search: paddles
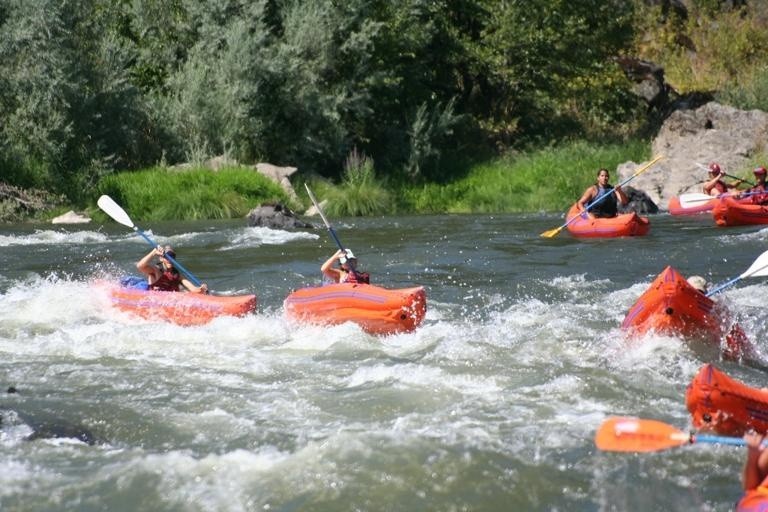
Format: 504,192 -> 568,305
696,163 -> 756,186
540,156 -> 663,238
594,416 -> 768,453
679,190 -> 767,209
98,194 -> 210,294
304,182 -> 358,276
701,251 -> 768,296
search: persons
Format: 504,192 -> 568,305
701,162 -> 745,198
743,429 -> 768,489
136,244 -> 209,294
578,168 -> 629,219
321,248 -> 369,285
685,275 -> 708,297
734,166 -> 768,205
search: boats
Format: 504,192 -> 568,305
93,278 -> 258,326
719,323 -> 768,374
566,200 -> 651,242
618,266 -> 730,339
283,281 -> 428,335
713,194 -> 768,229
685,363 -> 768,439
736,474 -> 768,512
668,189 -> 747,215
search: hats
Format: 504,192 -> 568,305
339,248 -> 357,265
752,167 -> 767,176
687,275 -> 707,292
708,163 -> 720,172
161,244 -> 177,257
